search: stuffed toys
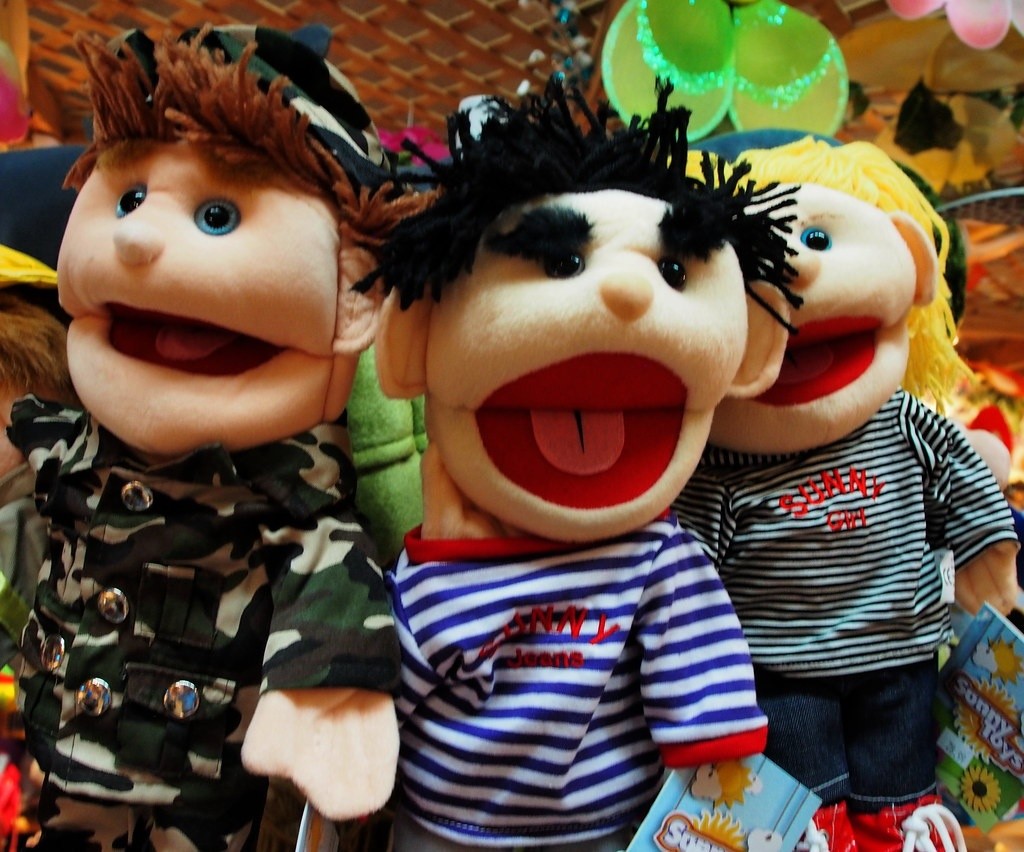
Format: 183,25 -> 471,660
0,21 -> 805,852
668,134 -> 1024,852
